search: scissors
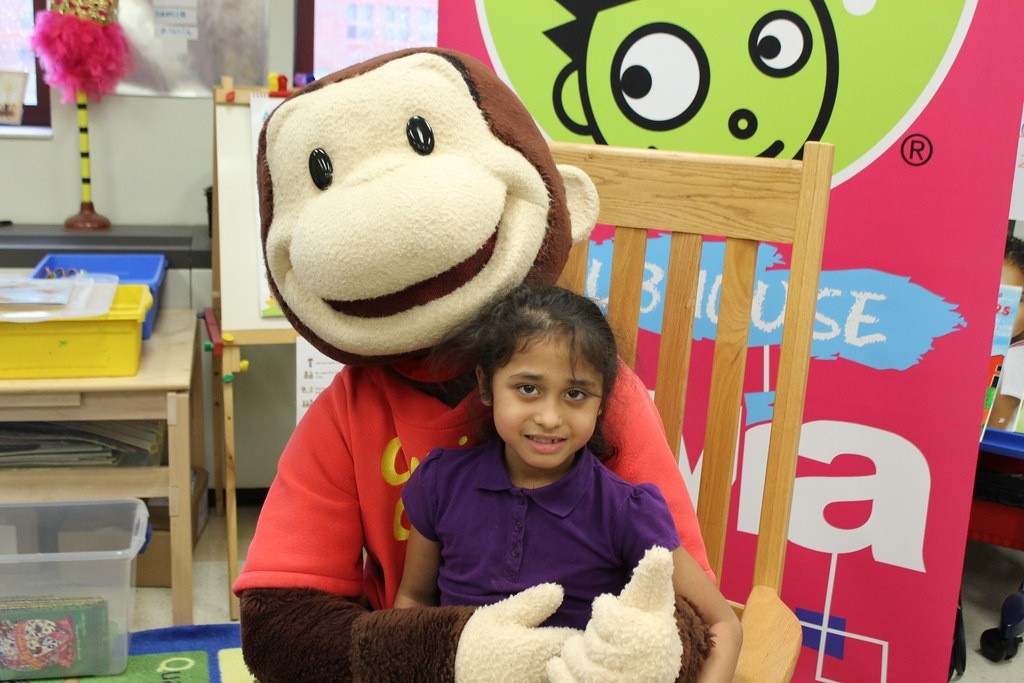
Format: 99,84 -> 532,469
55,267 -> 78,280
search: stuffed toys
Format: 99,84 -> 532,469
232,46 -> 719,683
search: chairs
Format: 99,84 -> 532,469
546,139 -> 835,683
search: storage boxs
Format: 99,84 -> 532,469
28,253 -> 169,340
0,464 -> 210,588
0,497 -> 152,682
0,283 -> 155,380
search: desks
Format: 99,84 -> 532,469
0,306 -> 205,627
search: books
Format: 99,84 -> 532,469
0,420 -> 164,470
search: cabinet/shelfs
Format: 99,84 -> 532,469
0,225 -> 296,489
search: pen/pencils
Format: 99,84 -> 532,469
46,267 -> 55,279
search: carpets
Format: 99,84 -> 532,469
2,623 -> 258,683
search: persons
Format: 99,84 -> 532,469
394,287 -> 742,683
